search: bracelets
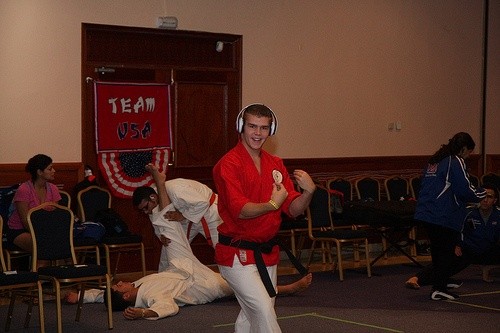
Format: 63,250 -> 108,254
142,309 -> 144,318
269,200 -> 279,210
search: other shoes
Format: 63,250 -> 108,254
430,290 -> 460,301
448,281 -> 463,289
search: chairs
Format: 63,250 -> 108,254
280,175 -> 500,281
0,183 -> 147,333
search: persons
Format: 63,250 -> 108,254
214,103 -> 315,332
6,154 -> 64,303
66,162 -> 315,321
133,178 -> 223,271
405,132 -> 500,299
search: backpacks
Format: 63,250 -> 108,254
73,218 -> 106,246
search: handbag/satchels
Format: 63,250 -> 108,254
95,207 -> 128,236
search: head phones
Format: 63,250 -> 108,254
235,103 -> 279,137
